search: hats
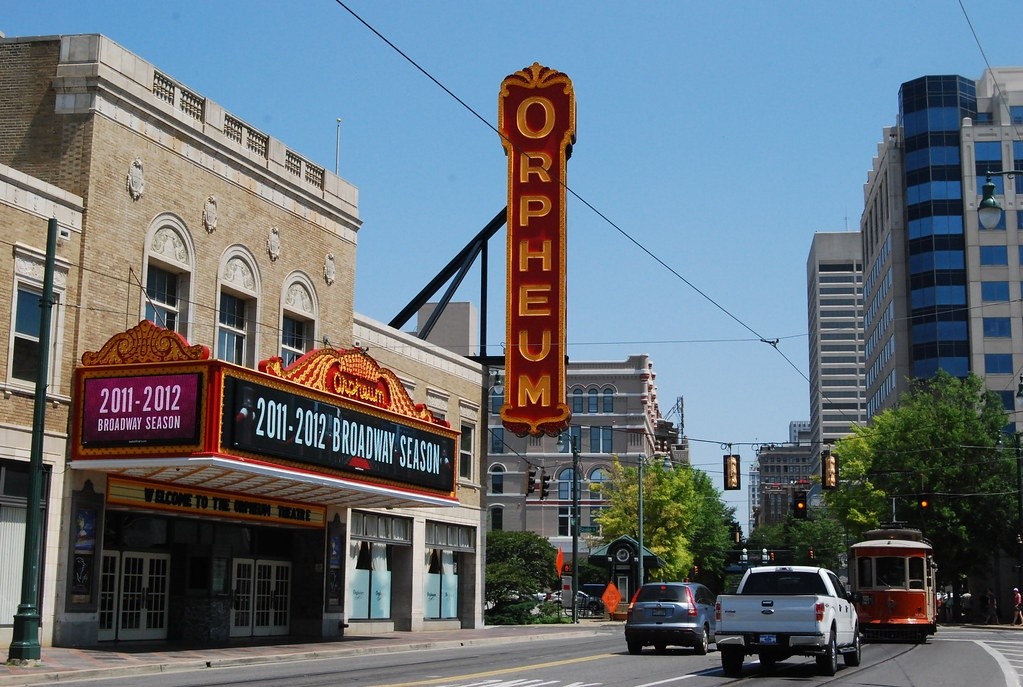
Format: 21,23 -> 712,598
1012,588 -> 1019,593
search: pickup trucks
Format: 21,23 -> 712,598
714,564 -> 867,673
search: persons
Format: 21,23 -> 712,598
1011,588 -> 1023,626
984,588 -> 999,624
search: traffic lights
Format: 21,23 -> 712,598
809,550 -> 815,560
564,565 -> 570,572
770,553 -> 777,562
792,490 -> 813,521
524,469 -> 537,498
919,492 -> 936,520
539,474 -> 551,501
693,566 -> 699,576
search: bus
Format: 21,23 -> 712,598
850,497 -> 940,642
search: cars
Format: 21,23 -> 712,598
548,589 -> 607,608
625,580 -> 716,651
506,590 -> 548,603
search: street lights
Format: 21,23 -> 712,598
995,430 -> 1023,597
638,453 -> 675,588
556,432 -> 582,623
